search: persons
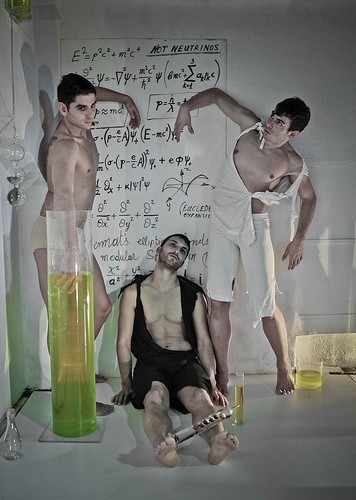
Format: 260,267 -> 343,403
174,86 -> 317,395
111,234 -> 240,468
30,73 -> 141,417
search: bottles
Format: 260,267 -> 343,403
4,409 -> 22,460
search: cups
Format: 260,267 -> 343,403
297,356 -> 324,389
